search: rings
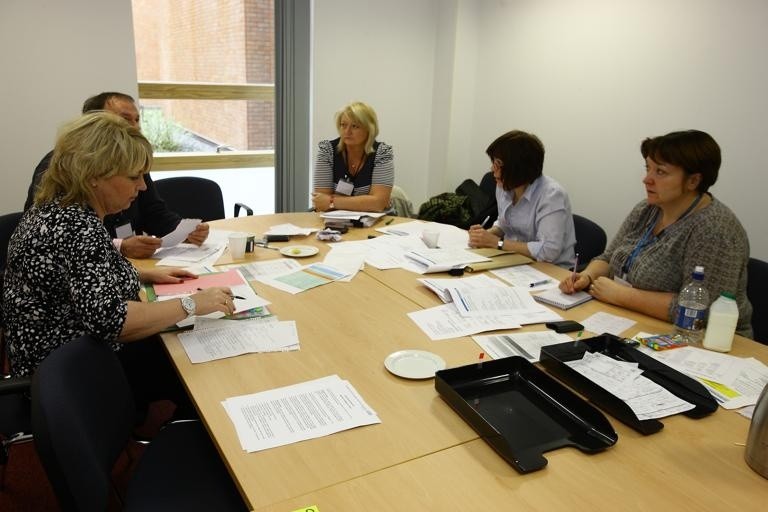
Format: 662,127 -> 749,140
591,284 -> 595,289
224,299 -> 228,305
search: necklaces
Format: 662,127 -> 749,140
351,164 -> 357,168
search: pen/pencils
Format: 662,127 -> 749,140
480,216 -> 490,228
254,239 -> 280,250
572,253 -> 579,295
529,279 -> 552,287
385,219 -> 394,225
197,288 -> 245,299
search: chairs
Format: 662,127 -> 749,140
571,212 -> 607,278
128,178 -> 254,238
744,256 -> 768,347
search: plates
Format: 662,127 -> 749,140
280,245 -> 319,258
383,350 -> 446,379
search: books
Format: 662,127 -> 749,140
533,286 -> 594,310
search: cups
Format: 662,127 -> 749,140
228,233 -> 247,258
422,229 -> 439,248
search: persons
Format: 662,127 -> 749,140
310,102 -> 395,212
467,130 -> 578,270
559,129 -> 756,339
22,91 -> 210,262
3,110 -> 240,378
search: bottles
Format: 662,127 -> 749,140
744,382 -> 767,478
702,289 -> 739,356
673,265 -> 708,344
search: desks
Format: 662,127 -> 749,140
134,210 -> 768,512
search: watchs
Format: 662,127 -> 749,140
178,295 -> 196,320
497,237 -> 504,250
329,194 -> 335,211
